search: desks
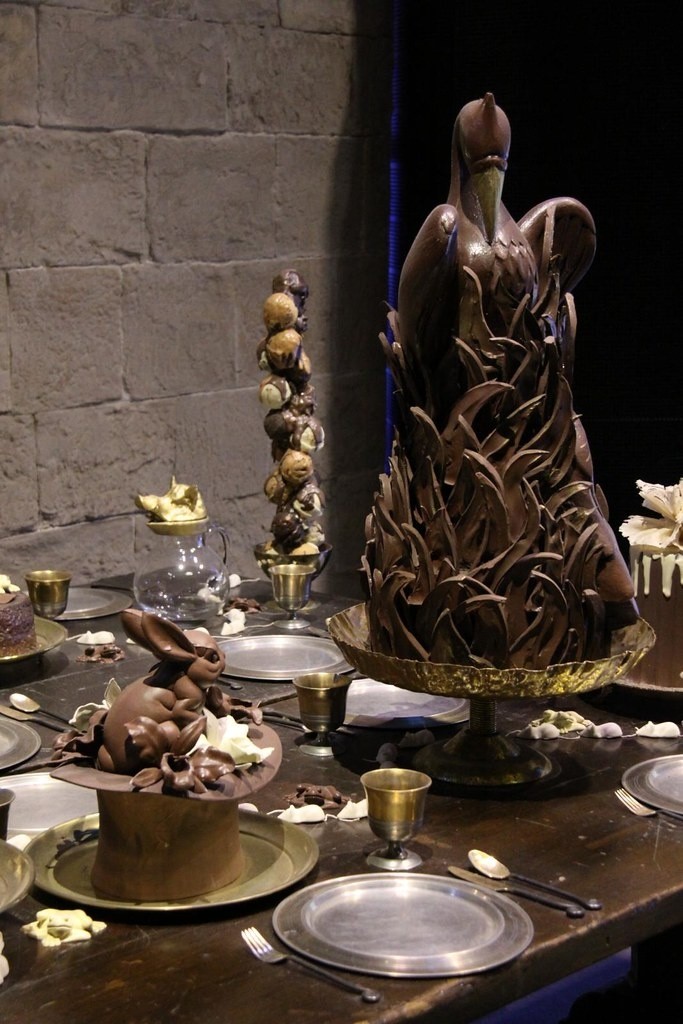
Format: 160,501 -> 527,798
1,571 -> 682,1024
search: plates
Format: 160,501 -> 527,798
343,676 -> 464,724
215,635 -> 355,681
272,872 -> 534,978
620,753 -> 683,813
0,715 -> 42,772
22,588 -> 132,621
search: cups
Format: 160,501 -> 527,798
23,569 -> 71,619
0,787 -> 16,840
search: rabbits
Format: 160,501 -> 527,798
97,609 -> 225,778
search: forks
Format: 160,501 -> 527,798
241,926 -> 381,1002
614,788 -> 683,821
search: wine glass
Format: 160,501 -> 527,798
292,672 -> 352,757
361,769 -> 432,871
269,564 -> 316,629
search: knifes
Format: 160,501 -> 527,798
0,704 -> 65,731
448,865 -> 585,917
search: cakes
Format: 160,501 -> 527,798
618,478 -> 683,689
0,574 -> 37,658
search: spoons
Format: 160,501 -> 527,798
10,693 -> 68,722
468,848 -> 602,909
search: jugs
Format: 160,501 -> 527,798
133,477 -> 231,622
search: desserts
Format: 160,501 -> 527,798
337,714 -> 434,768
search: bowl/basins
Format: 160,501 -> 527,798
0,618 -> 68,661
0,838 -> 36,914
